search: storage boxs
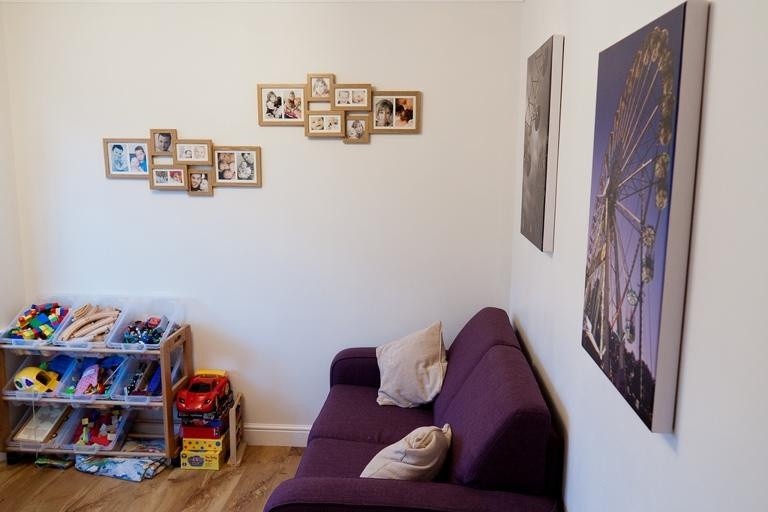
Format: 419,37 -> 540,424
52,298 -> 125,360
0,304 -> 73,356
1,353 -> 75,410
60,406 -> 138,453
112,350 -> 182,412
107,294 -> 181,360
3,405 -> 78,449
56,355 -> 127,409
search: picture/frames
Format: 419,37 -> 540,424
257,72 -> 422,144
102,129 -> 262,196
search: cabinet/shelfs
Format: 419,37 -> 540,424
0,323 -> 195,465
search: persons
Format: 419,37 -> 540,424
109,135 -> 255,192
266,79 -> 413,138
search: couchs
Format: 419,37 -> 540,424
262,307 -> 565,512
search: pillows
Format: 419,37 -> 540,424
376,319 -> 448,408
359,423 -> 452,482
